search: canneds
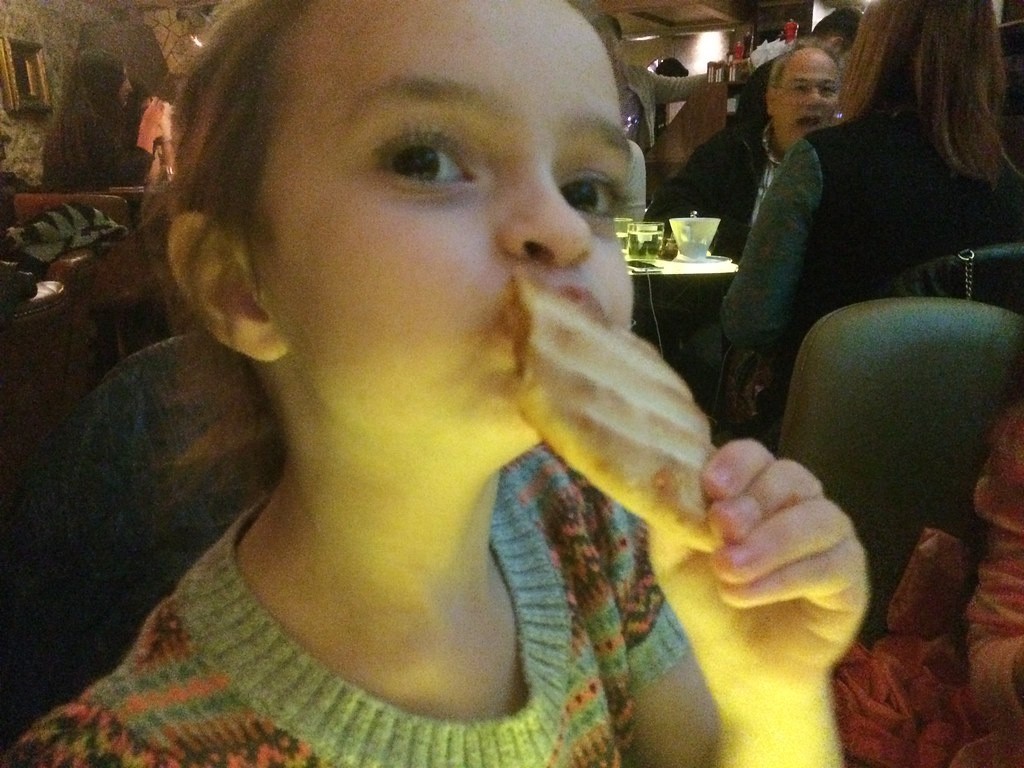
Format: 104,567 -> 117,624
707,61 -> 741,81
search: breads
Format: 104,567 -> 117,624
502,275 -> 723,553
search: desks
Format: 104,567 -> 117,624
631,235 -> 738,347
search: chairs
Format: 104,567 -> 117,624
772,290 -> 1024,651
0,190 -> 278,749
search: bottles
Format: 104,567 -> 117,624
734,41 -> 744,60
144,136 -> 173,193
784,18 -> 799,43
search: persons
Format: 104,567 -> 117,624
42,49 -> 165,187
592,15 -> 705,151
963,364 -> 1024,719
0,0 -> 870,767
721,1 -> 1024,352
734,8 -> 863,134
642,39 -> 843,261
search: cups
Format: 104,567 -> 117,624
708,60 -> 737,83
612,216 -> 634,254
626,222 -> 666,265
669,216 -> 721,260
